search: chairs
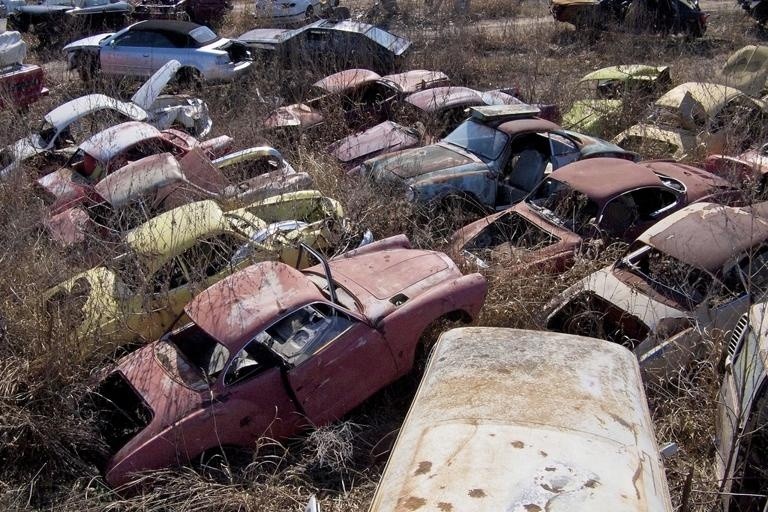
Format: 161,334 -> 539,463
499,150 -> 545,202
